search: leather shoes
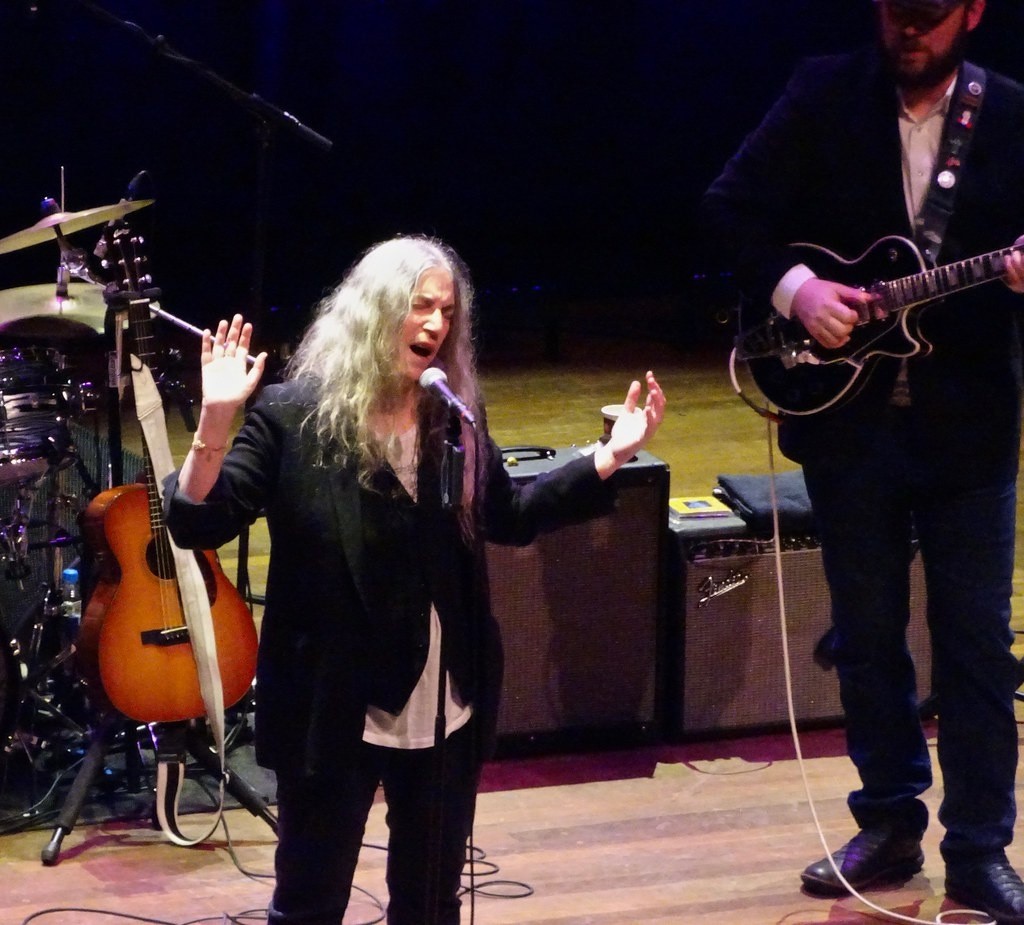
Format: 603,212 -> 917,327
941,848 -> 1024,924
797,816 -> 926,895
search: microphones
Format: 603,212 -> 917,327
418,367 -> 476,424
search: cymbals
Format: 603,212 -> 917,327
0,199 -> 156,255
0,282 -> 160,334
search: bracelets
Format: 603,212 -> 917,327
191,431 -> 230,462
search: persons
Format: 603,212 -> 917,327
670,0 -> 1024,925
162,235 -> 665,925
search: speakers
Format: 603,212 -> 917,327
480,446 -> 933,755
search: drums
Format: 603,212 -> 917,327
1,346 -> 79,487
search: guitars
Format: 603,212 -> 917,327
737,237 -> 1023,417
73,214 -> 260,724
